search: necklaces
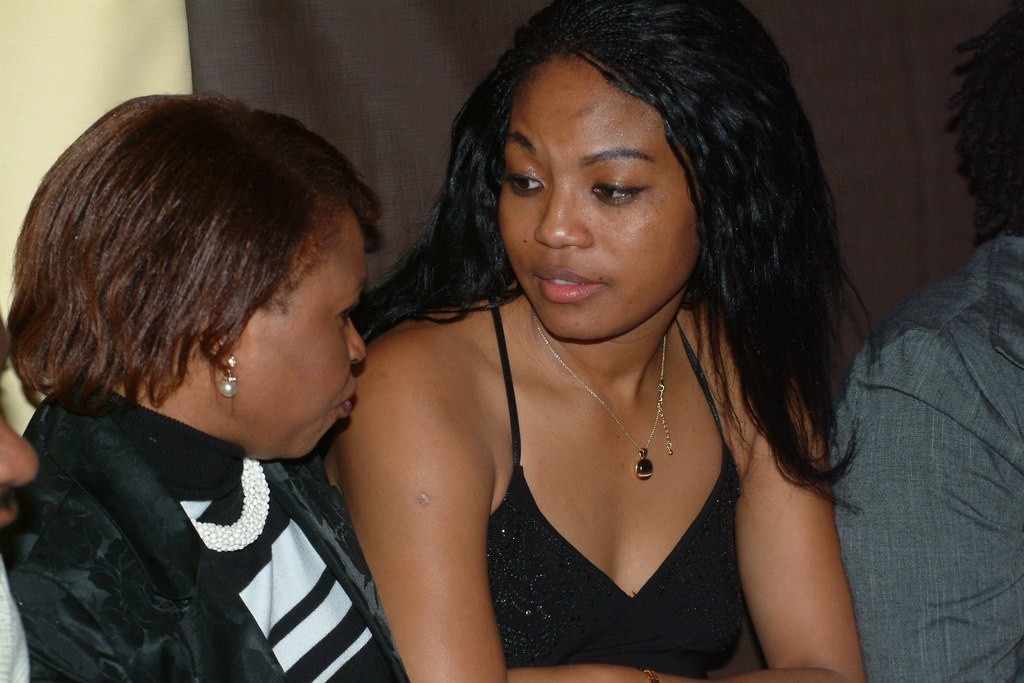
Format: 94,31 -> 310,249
532,313 -> 675,481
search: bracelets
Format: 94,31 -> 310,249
639,666 -> 661,683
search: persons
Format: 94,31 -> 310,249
0,315 -> 50,683
820,0 -> 1024,683
12,92 -> 417,683
307,0 -> 874,683
